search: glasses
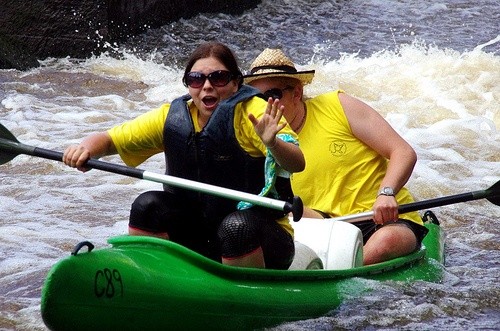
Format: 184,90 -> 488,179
184,70 -> 236,89
260,86 -> 293,102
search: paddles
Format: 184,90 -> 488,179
329,179 -> 500,222
0,123 -> 304,221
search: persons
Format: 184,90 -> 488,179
61,42 -> 306,270
242,47 -> 429,265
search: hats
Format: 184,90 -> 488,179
243,48 -> 315,86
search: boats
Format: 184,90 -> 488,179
39,209 -> 448,331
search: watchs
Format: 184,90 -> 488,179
376,186 -> 396,198
